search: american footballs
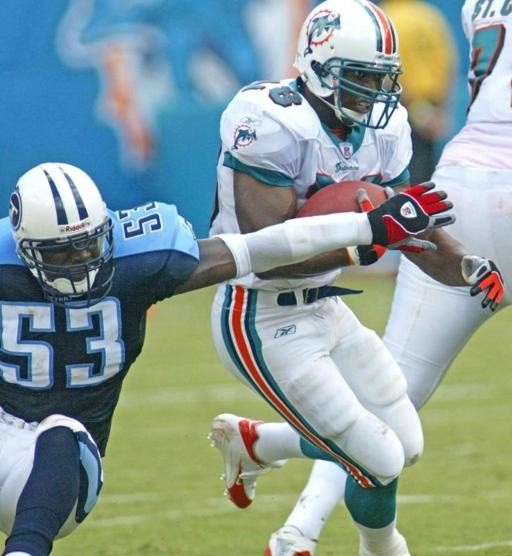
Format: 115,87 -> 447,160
294,181 -> 387,218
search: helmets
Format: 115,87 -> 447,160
294,0 -> 399,98
10,163 -> 108,297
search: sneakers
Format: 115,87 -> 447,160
265,533 -> 316,556
208,414 -> 287,509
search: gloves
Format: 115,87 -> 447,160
461,255 -> 505,312
346,180 -> 456,265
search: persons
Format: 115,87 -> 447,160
1,161 -> 455,556
211,1 -> 503,556
264,1 -> 512,555
